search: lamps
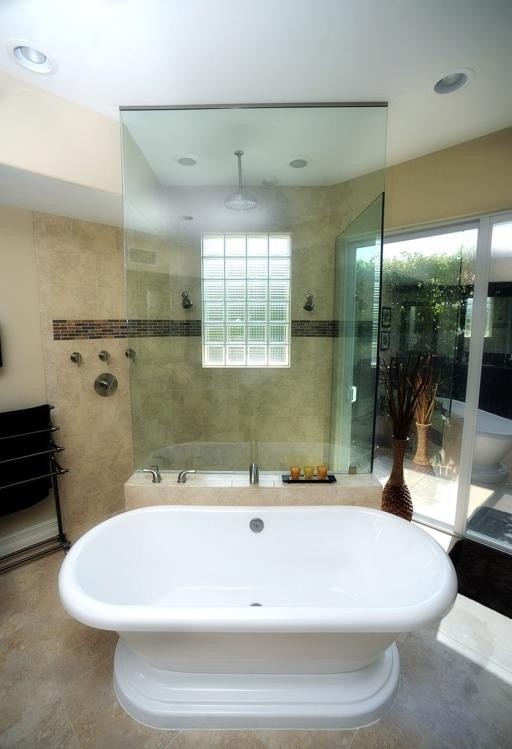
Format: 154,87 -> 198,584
224,138 -> 258,212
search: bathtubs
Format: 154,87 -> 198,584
142,441 -> 372,475
434,396 -> 512,483
59,504 -> 458,734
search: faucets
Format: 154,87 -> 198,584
249,464 -> 259,484
249,437 -> 258,463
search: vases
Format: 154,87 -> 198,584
380,441 -> 415,522
